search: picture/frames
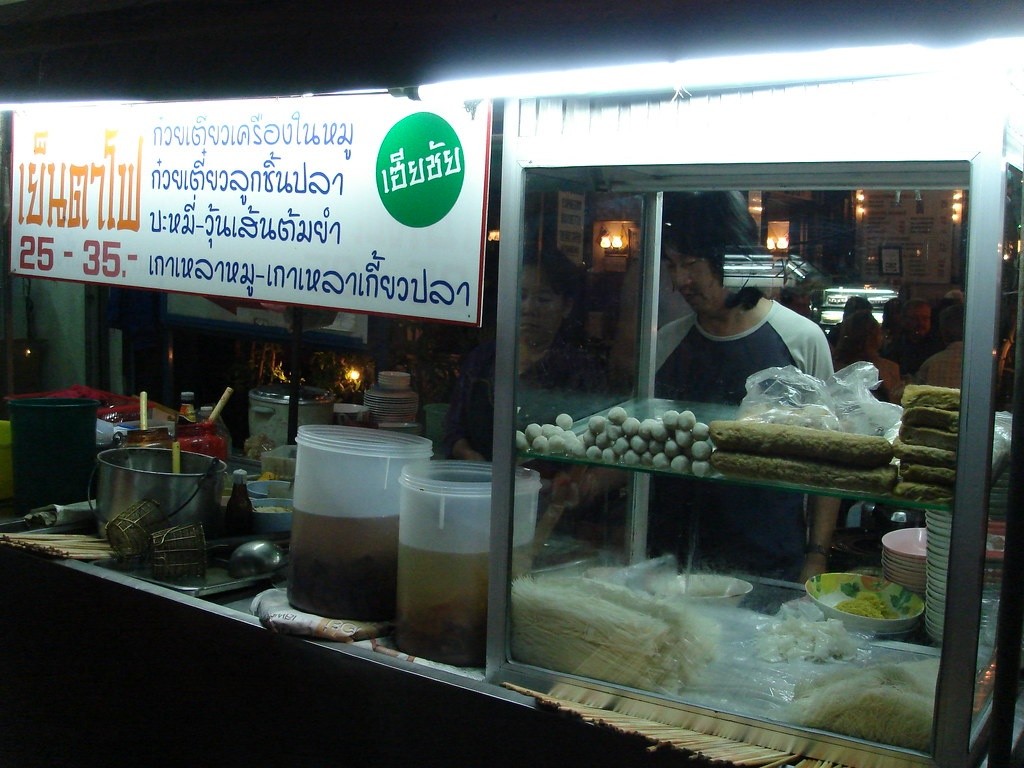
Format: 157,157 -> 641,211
878,246 -> 903,277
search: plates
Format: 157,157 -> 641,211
333,371 -> 419,434
880,472 -> 1009,648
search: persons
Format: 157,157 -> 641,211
439,191 -> 964,587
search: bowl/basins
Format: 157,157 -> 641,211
251,498 -> 293,533
246,481 -> 293,499
807,573 -> 924,633
664,575 -> 753,608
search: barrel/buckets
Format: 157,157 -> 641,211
288,425 -> 434,621
7,397 -> 100,514
423,404 -> 449,459
396,459 -> 541,667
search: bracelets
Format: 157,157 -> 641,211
804,543 -> 830,557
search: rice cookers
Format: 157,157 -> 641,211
248,383 -> 334,447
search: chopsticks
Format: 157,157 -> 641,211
501,682 -> 850,768
0,533 -> 118,559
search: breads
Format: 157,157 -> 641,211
708,383 -> 961,503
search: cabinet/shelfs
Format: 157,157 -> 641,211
484,75 -> 1024,768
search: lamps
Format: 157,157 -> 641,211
601,235 -> 611,254
612,235 -> 623,253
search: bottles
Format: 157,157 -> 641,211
126,427 -> 173,449
175,423 -> 224,459
225,469 -> 253,537
179,392 -> 195,425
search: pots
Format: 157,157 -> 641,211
88,447 -> 227,539
